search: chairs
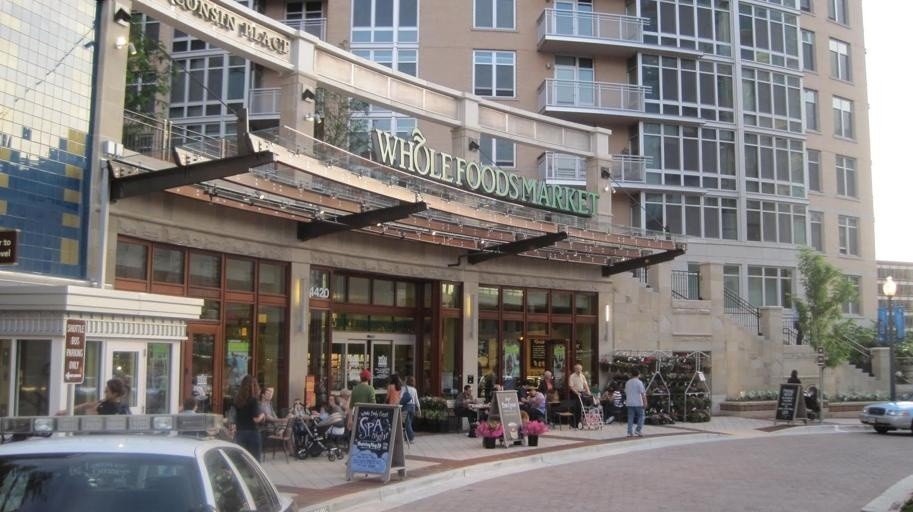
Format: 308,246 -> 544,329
257,410 -> 412,465
455,396 -> 626,431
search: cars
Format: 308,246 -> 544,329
858,394 -> 912,434
0,432 -> 299,512
71,375 -> 168,412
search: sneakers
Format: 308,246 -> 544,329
626,429 -> 643,440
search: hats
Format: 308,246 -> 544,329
358,368 -> 371,379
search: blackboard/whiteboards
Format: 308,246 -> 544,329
776,383 -> 800,422
347,403 -> 399,475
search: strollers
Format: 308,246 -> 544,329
294,412 -> 346,463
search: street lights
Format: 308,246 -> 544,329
882,274 -> 899,402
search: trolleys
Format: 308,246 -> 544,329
576,392 -> 604,432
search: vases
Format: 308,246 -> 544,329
413,416 -> 449,433
528,435 -> 538,446
483,437 -> 495,448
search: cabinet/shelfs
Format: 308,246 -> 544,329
598,350 -> 712,424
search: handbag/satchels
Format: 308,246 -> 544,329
400,387 -> 412,407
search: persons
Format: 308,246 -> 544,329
233,376 -> 265,464
400,376 -> 421,442
454,386 -> 479,438
384,374 -> 402,404
259,386 -> 356,457
177,398 -> 219,437
625,369 -> 647,437
788,370 -> 801,383
518,364 -> 624,429
347,371 -> 376,429
56,379 -> 130,415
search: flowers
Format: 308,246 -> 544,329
473,419 -> 503,439
416,395 -> 448,419
518,420 -> 550,435
